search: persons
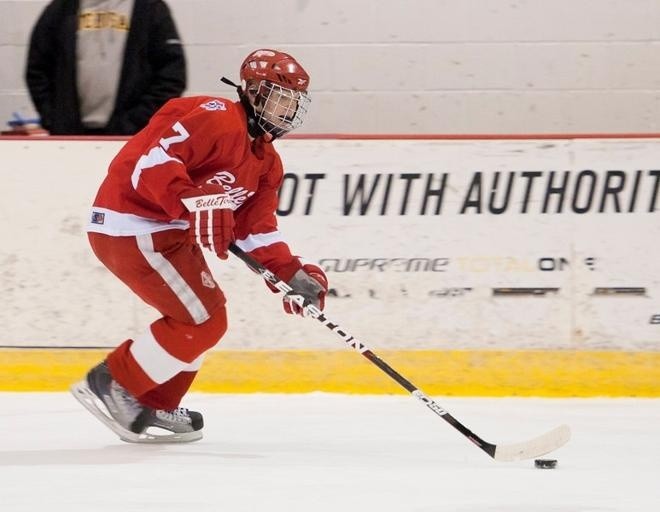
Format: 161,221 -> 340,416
69,50 -> 330,441
24,0 -> 188,136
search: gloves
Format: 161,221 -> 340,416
278,263 -> 329,317
189,181 -> 238,263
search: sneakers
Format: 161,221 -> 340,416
150,407 -> 204,433
85,358 -> 150,435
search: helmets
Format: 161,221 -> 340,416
237,47 -> 311,101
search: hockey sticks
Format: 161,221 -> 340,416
228,241 -> 572,464
